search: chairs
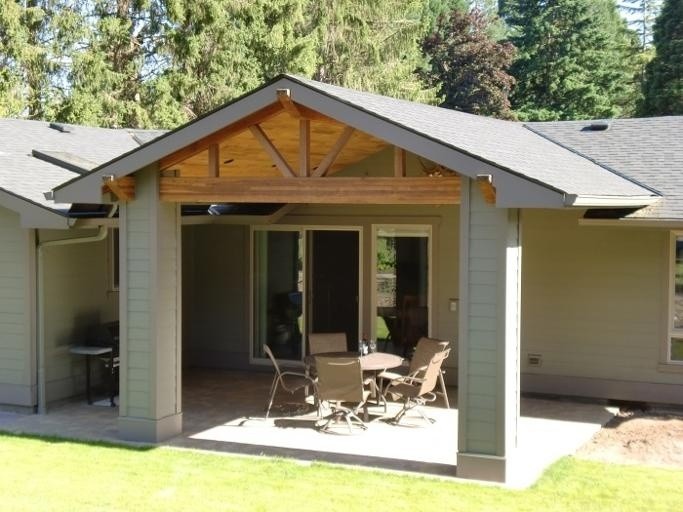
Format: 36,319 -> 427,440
262,332 -> 452,434
